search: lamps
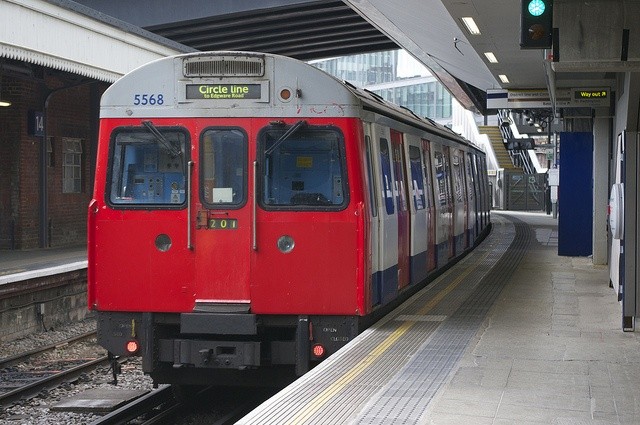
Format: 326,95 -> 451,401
524,111 -> 544,133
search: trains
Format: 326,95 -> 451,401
85,51 -> 492,396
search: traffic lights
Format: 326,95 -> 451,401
522,1 -> 555,48
